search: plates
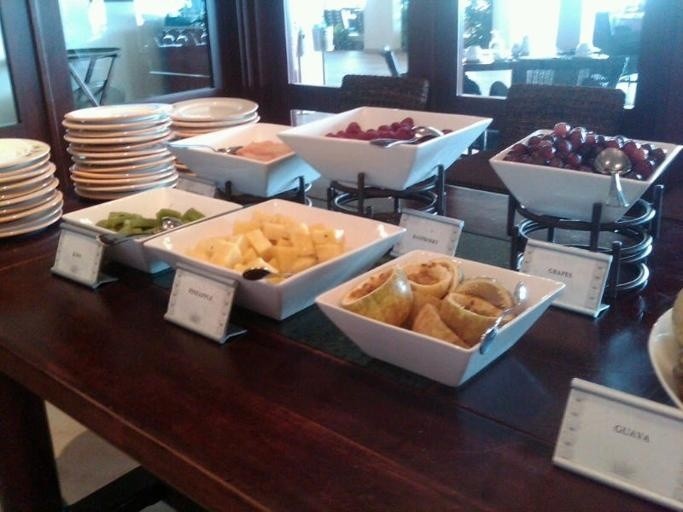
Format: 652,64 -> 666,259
1,94 -> 261,239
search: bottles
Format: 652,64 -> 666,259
511,36 -> 530,56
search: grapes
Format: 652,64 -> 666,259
503,121 -> 666,180
324,115 -> 452,141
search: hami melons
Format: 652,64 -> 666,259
185,206 -> 350,281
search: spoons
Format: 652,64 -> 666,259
97,219 -> 176,248
371,125 -> 443,149
479,281 -> 528,355
594,147 -> 633,209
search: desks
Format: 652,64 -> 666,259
62,47 -> 119,107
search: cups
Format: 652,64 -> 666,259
466,45 -> 482,61
575,43 -> 593,57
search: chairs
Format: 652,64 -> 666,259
341,41 -> 629,153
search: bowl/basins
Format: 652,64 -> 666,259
488,127 -> 683,224
162,121 -> 322,197
312,248 -> 567,388
142,196 -> 408,322
59,185 -> 245,275
276,104 -> 494,194
